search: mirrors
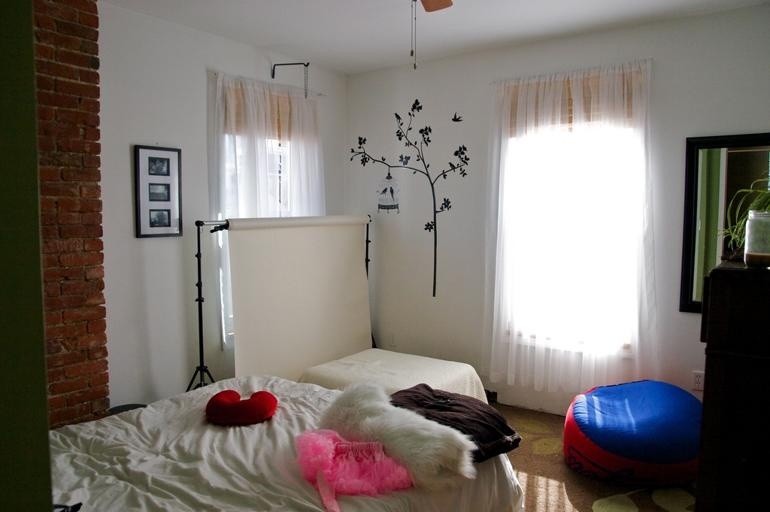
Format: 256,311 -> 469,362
675,130 -> 770,315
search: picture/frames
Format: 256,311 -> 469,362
132,144 -> 185,240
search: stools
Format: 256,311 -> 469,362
293,343 -> 487,425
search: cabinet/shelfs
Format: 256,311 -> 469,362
694,254 -> 770,511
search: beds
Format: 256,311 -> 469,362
42,369 -> 526,512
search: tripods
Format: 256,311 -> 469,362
186,226 -> 216,393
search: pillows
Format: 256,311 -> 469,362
203,388 -> 279,427
